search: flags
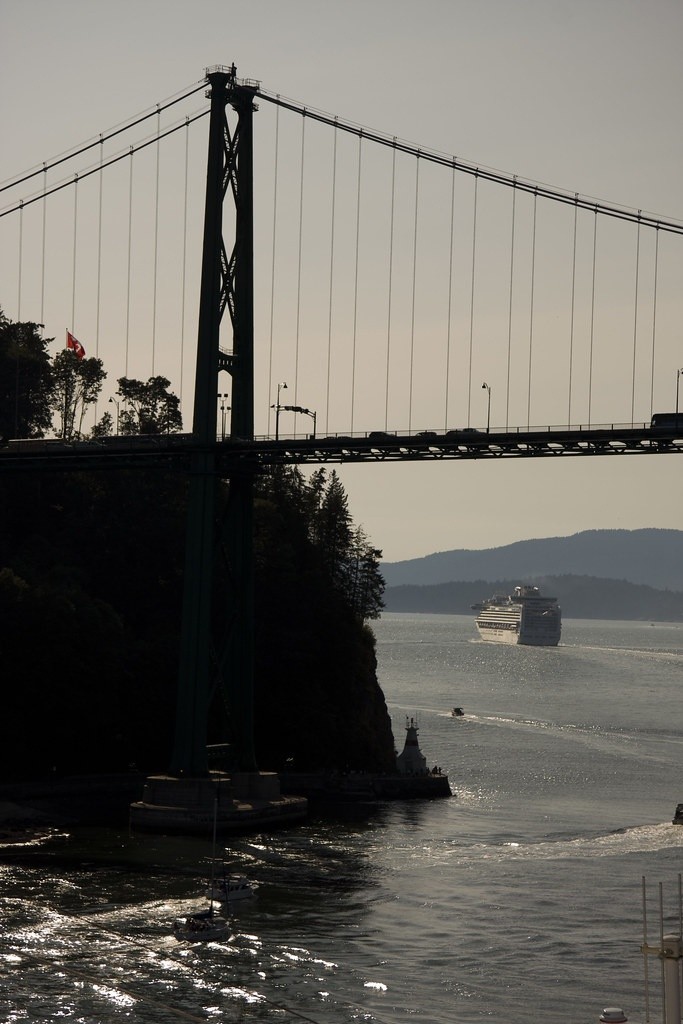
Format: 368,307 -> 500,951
67,332 -> 85,358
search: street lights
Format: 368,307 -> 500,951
276,382 -> 288,441
481,382 -> 491,435
217,394 -> 231,441
109,397 -> 119,436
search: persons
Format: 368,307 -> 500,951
428,765 -> 441,776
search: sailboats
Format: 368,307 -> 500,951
173,796 -> 229,941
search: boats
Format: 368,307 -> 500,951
130,794 -> 308,833
451,706 -> 464,717
205,872 -> 259,902
470,584 -> 562,646
